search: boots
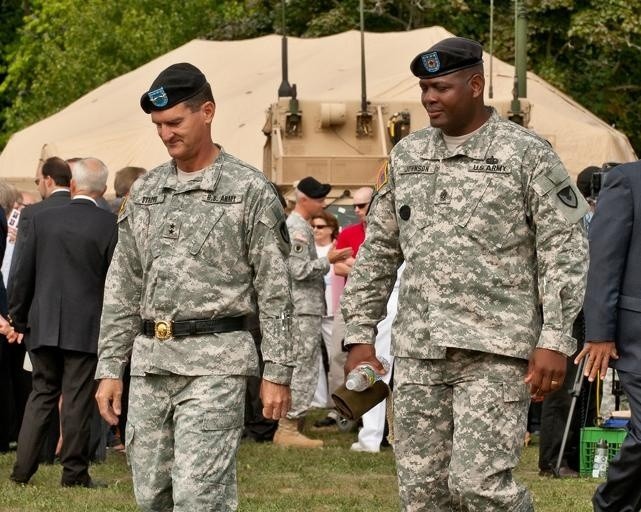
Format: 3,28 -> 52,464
273,415 -> 324,450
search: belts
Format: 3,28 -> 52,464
136,313 -> 251,343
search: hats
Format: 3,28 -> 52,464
408,34 -> 487,78
575,162 -> 623,200
296,175 -> 333,200
140,59 -> 210,117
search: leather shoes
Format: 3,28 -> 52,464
540,459 -> 580,479
62,473 -> 111,491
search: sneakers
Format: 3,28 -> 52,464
349,442 -> 382,453
315,415 -> 337,427
107,435 -> 124,451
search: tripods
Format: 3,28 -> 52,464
552,342 -> 601,478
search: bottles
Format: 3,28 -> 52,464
592,438 -> 609,479
345,355 -> 391,392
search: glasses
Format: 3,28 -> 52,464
312,223 -> 331,229
352,202 -> 370,209
34,175 -> 46,186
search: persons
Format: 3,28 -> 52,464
524,156 -> 641,512
1,154 -> 406,491
91,60 -> 296,512
334,35 -> 592,512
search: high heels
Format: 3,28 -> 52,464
524,438 -> 532,448
